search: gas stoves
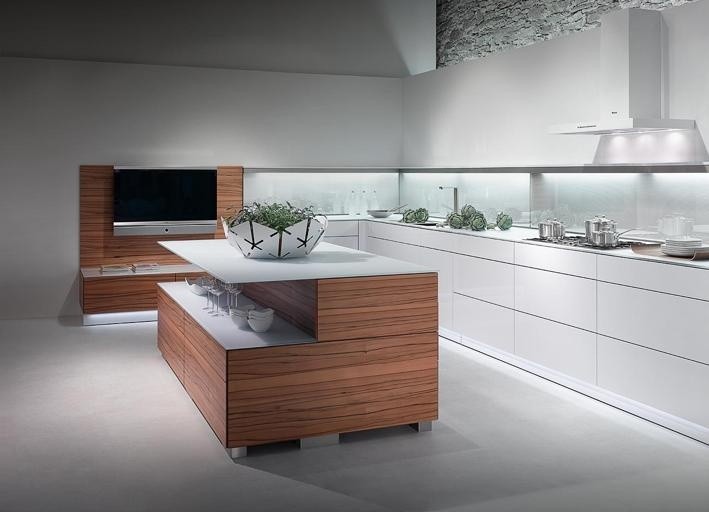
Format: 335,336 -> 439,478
526,235 -> 646,252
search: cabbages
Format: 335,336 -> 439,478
448,204 -> 513,231
402,208 -> 429,223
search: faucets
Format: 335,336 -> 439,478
439,186 -> 458,213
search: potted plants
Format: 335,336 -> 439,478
221,198 -> 325,260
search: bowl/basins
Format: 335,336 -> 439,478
367,210 -> 392,219
229,301 -> 276,334
184,276 -> 212,295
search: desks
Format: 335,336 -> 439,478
155,238 -> 440,460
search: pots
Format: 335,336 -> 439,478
538,217 -> 566,240
582,215 -> 640,247
658,213 -> 697,237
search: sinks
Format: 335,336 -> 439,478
414,223 -> 448,226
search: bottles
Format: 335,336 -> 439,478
349,190 -> 358,216
359,191 -> 368,215
370,189 -> 379,210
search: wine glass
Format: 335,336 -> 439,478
200,273 -> 243,315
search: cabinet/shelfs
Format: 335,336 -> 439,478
325,222 -> 359,251
80,264 -> 214,326
440,231 -> 514,356
366,221 -> 439,301
515,241 -> 597,391
597,253 -> 708,430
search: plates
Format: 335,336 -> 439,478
658,238 -> 709,260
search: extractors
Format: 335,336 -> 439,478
543,9 -> 709,136
